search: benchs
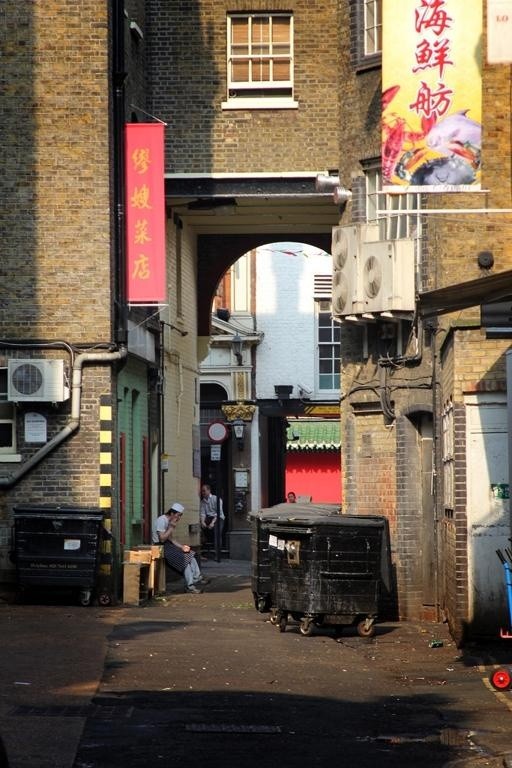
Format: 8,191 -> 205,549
164,544 -> 201,583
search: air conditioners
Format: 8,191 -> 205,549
330,222 -> 415,324
7,358 -> 70,403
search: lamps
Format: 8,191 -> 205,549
232,414 -> 246,451
232,330 -> 243,366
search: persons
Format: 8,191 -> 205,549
151,503 -> 210,594
200,485 -> 225,544
288,492 -> 295,502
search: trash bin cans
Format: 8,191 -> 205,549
252,502 -> 387,636
11,505 -> 102,608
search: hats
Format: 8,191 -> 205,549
171,502 -> 185,514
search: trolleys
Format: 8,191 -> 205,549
486,545 -> 512,692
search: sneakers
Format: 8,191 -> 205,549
184,584 -> 203,594
194,578 -> 211,586
